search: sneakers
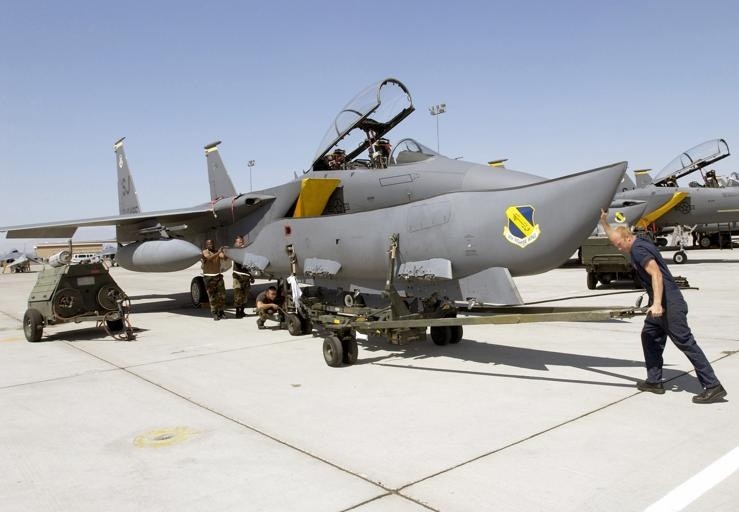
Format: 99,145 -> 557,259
219,310 -> 229,319
212,311 -> 221,321
235,311 -> 243,318
636,376 -> 666,395
256,318 -> 266,329
692,383 -> 728,404
240,309 -> 248,317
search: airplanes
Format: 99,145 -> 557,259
588,138 -> 739,264
2,78 -> 631,366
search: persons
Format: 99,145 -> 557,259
255,285 -> 289,330
325,148 -> 348,170
200,238 -> 228,321
702,170 -> 719,188
599,207 -> 728,405
368,138 -> 396,167
231,234 -> 257,319
